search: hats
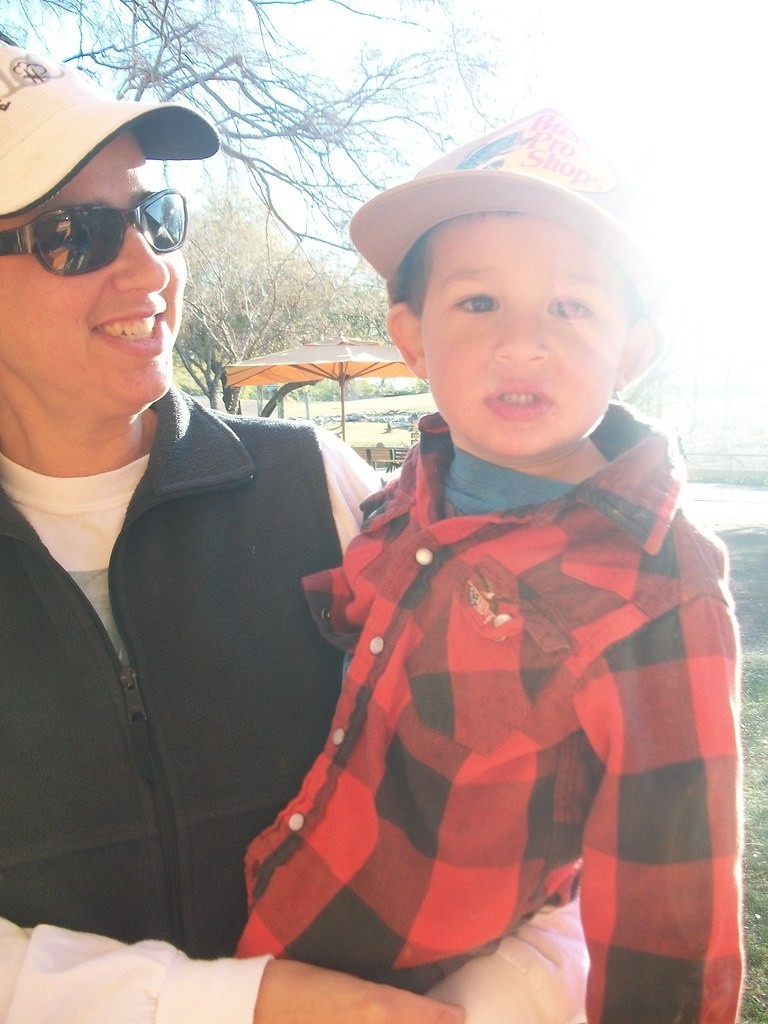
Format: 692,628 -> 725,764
348,108 -> 656,323
0,40 -> 220,219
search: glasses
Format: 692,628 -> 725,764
0,189 -> 188,276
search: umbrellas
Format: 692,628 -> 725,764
222,333 -> 420,440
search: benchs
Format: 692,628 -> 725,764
352,443 -> 409,473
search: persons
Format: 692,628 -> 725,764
0,44 -> 589,1024
235,106 -> 745,1024
